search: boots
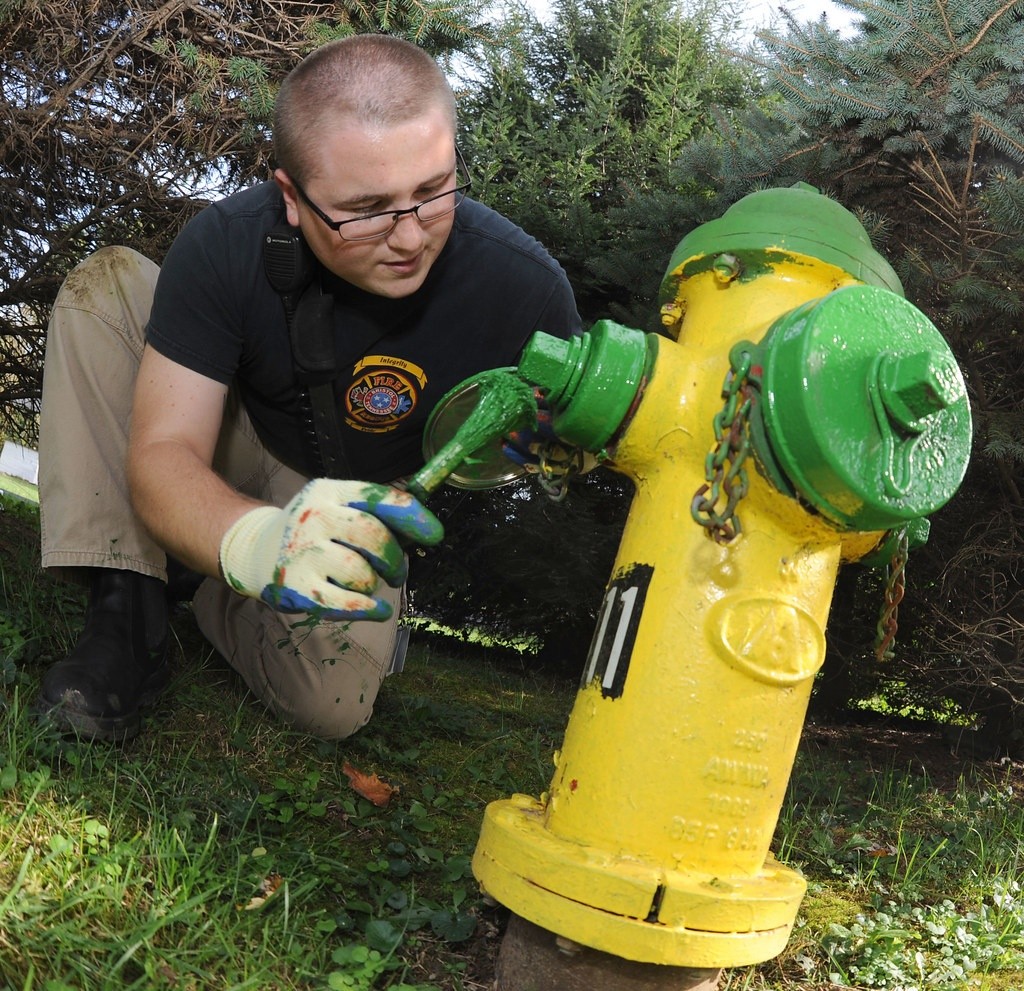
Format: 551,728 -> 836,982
37,565 -> 169,740
161,551 -> 207,600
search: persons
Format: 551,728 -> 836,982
36,36 -> 583,742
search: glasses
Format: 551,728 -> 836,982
277,145 -> 476,241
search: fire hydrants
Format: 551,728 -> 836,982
420,175 -> 975,990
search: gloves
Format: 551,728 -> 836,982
219,471 -> 445,622
497,387 -> 607,476
